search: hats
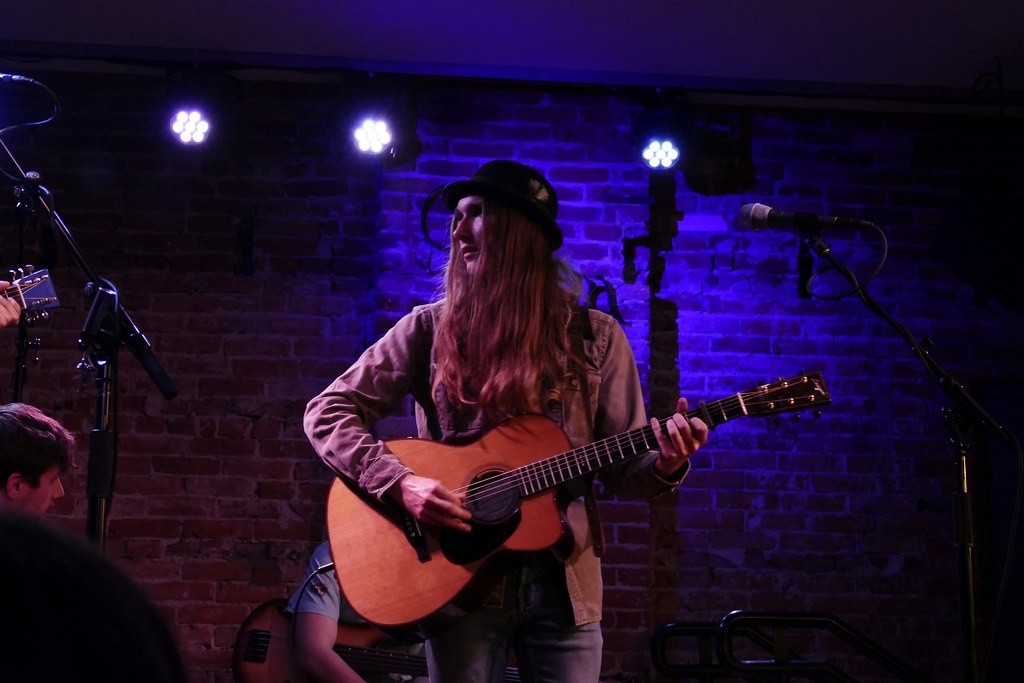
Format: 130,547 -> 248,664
441,160 -> 564,257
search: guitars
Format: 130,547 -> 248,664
325,368 -> 833,643
238,600 -> 647,682
1,267 -> 66,321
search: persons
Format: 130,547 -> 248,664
1,280 -> 194,682
287,540 -> 430,683
303,161 -> 708,683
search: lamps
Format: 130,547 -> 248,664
632,112 -> 680,172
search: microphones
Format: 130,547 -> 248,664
0,73 -> 35,85
739,203 -> 874,230
